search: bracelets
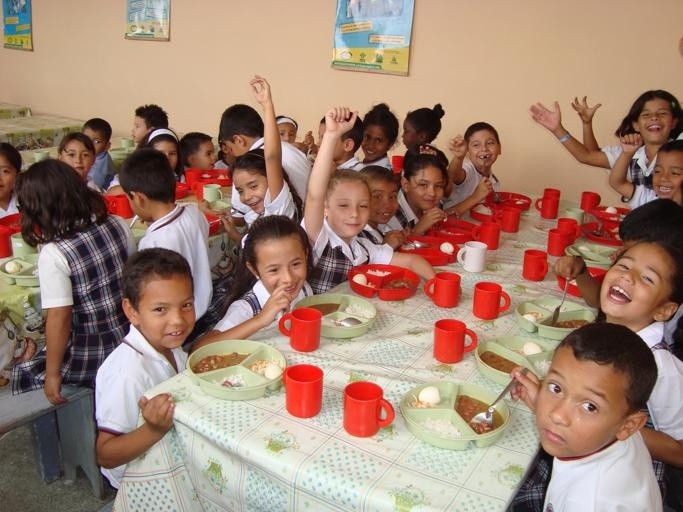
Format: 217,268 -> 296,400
577,254 -> 587,277
559,132 -> 571,147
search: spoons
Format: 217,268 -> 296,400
492,190 -> 499,203
552,275 -> 569,324
469,367 -> 529,426
407,241 -> 429,248
322,317 -> 362,327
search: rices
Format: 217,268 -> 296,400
418,418 -> 463,441
209,373 -> 244,389
472,204 -> 494,216
535,359 -> 551,376
367,268 -> 391,276
541,303 -> 566,313
402,240 -> 419,251
344,304 -> 374,319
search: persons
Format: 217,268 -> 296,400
402,103 -> 449,170
215,139 -> 236,168
403,142 -> 494,214
218,104 -> 312,210
361,103 -> 401,165
189,216 -> 313,351
316,112 -> 364,171
530,90 -> 683,188
570,95 -> 602,151
599,238 -> 683,468
451,122 -> 501,206
179,132 -> 216,186
671,182 -> 683,204
358,164 -> 407,253
209,78 -> 301,319
96,247 -> 195,490
106,128 -> 191,216
119,149 -> 213,347
388,152 -> 461,238
510,322 -> 663,507
56,133 -> 104,196
299,107 -> 437,296
276,114 -> 314,155
82,119 -> 116,191
553,198 -> 683,308
21,158 -> 137,405
608,133 -> 683,210
132,105 -> 169,149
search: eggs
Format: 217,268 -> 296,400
524,342 -> 543,357
321,321 -> 335,326
353,274 -> 367,285
606,207 -> 618,213
579,246 -> 591,252
440,242 -> 455,255
419,387 -> 442,405
265,365 -> 281,379
6,262 -> 21,274
524,313 -> 535,324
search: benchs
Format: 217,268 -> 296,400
1,384 -> 103,500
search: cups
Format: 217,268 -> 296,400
543,189 -> 560,199
457,241 -> 488,273
432,318 -> 478,365
565,208 -> 584,225
190,181 -> 210,199
497,206 -> 519,232
557,218 -> 582,245
10,231 -> 38,257
282,365 -> 324,418
424,272 -> 460,308
473,282 -> 511,320
109,193 -> 134,218
202,184 -> 224,203
547,229 -> 567,257
534,197 -> 559,219
278,307 -> 320,350
523,250 -> 548,281
392,155 -> 404,175
343,382 -> 395,439
580,192 -> 601,212
472,223 -> 499,250
185,169 -> 201,186
